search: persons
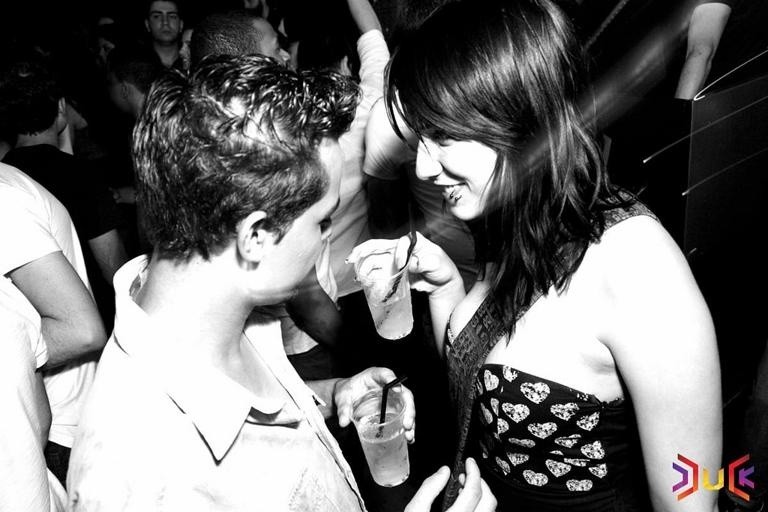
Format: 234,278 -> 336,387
0,0 -> 768,511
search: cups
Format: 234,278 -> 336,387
351,390 -> 410,488
355,248 -> 414,340
